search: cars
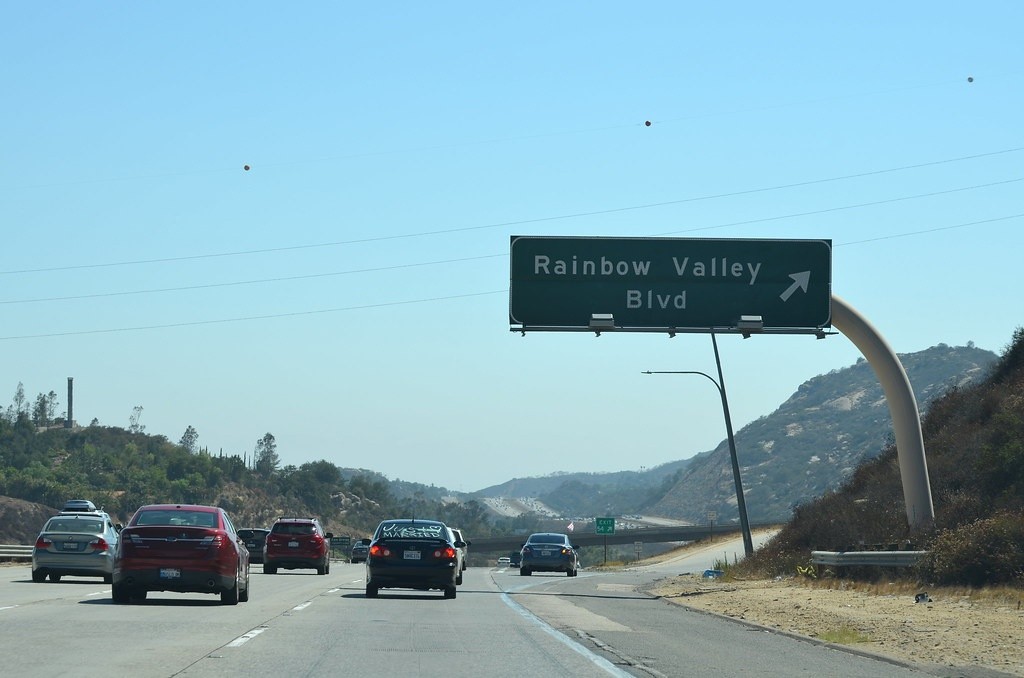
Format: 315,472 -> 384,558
510,551 -> 520,568
497,557 -> 510,567
31,515 -> 120,585
519,533 -> 580,577
447,525 -> 471,585
362,516 -> 466,599
112,504 -> 250,606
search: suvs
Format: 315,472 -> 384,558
352,540 -> 371,564
59,499 -> 110,517
264,516 -> 333,576
237,527 -> 271,565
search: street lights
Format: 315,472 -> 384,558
641,370 -> 755,557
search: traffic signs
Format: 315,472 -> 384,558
507,234 -> 833,337
595,517 -> 615,534
